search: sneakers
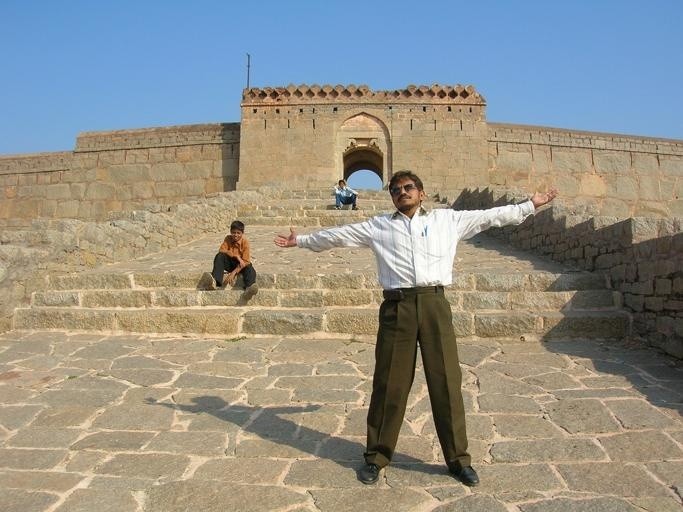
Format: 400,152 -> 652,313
208,276 -> 217,290
243,283 -> 258,300
334,205 -> 359,210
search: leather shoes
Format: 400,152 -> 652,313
455,466 -> 479,486
358,465 -> 379,484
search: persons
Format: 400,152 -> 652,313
205,221 -> 258,300
334,180 -> 359,210
274,171 -> 559,487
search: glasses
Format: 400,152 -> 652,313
391,184 -> 414,194
